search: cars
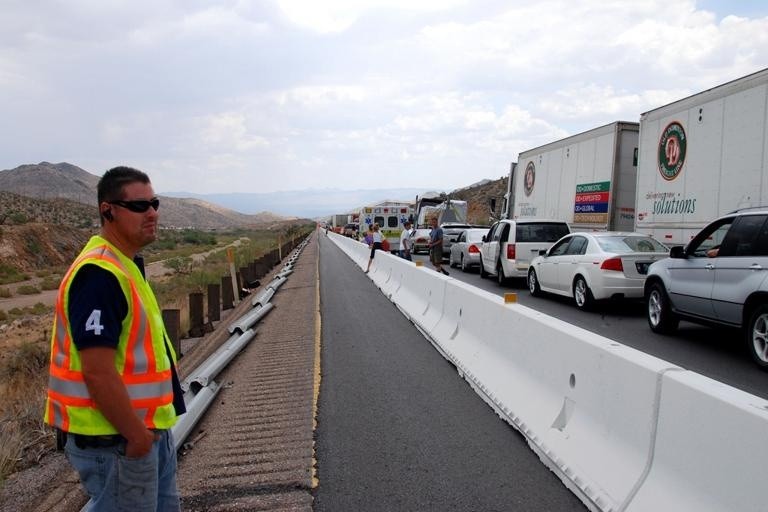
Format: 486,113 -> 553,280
526,230 -> 672,311
479,218 -> 570,286
327,196 -> 472,259
449,227 -> 491,272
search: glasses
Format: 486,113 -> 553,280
111,200 -> 161,212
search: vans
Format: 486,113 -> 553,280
644,202 -> 768,371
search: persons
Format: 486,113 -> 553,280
44,167 -> 187,511
400,221 -> 411,261
428,218 -> 444,271
351,223 -> 389,273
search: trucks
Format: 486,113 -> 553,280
488,120 -> 640,231
631,67 -> 768,251
